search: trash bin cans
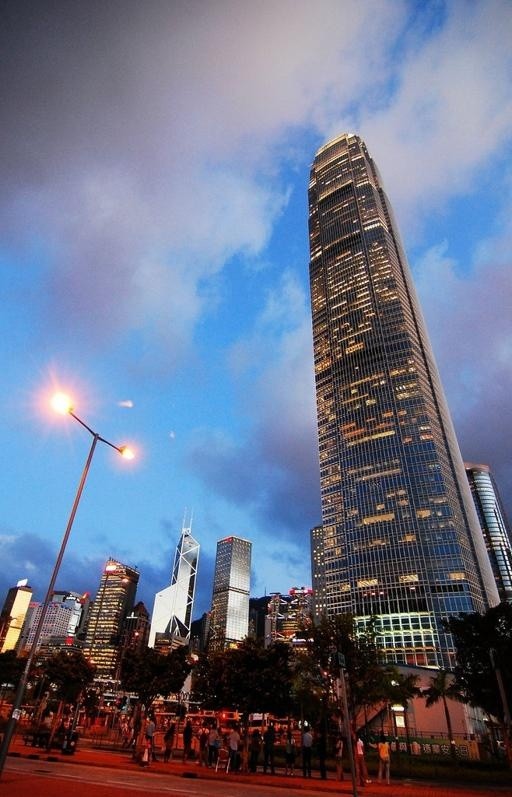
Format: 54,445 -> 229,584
62,731 -> 80,755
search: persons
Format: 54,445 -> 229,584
121,715 -> 392,785
33,710 -> 78,751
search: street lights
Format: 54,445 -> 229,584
0,394 -> 135,776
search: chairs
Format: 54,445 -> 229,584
213,748 -> 230,773
